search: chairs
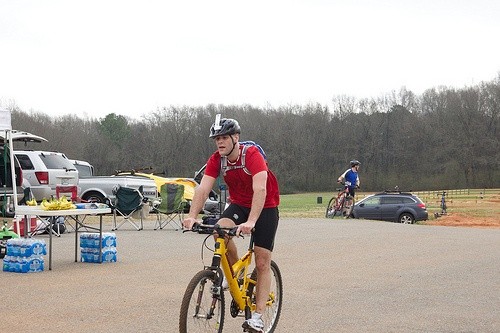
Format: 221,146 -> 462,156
25,183 -> 185,238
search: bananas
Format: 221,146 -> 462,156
41,195 -> 77,210
26,197 -> 37,206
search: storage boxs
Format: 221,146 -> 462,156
14,218 -> 36,236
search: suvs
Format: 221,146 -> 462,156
328,189 -> 428,225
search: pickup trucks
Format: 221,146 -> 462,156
69,159 -> 162,216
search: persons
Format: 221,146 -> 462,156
338,159 -> 361,215
183,118 -> 280,333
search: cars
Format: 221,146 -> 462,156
110,166 -> 223,214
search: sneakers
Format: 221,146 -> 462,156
242,313 -> 264,333
222,276 -> 229,290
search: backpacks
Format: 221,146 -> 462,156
220,141 -> 267,176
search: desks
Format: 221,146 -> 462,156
16,208 -> 112,270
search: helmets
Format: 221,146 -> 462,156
350,160 -> 361,166
209,118 -> 241,137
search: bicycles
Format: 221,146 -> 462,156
179,220 -> 284,333
325,181 -> 360,219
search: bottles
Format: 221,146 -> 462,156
2,238 -> 48,274
80,232 -> 118,264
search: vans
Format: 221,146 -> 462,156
0,127 -> 81,218
